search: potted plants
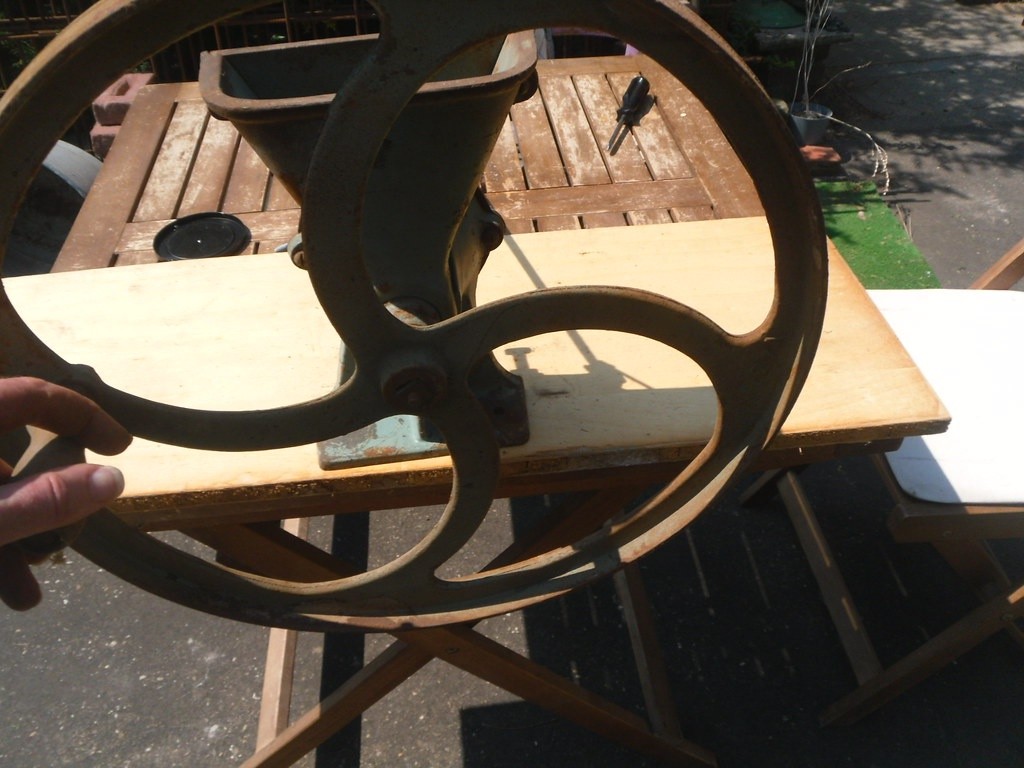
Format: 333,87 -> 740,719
785,0 -> 835,147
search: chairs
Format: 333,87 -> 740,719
734,234 -> 1024,734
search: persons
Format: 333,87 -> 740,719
0,377 -> 133,611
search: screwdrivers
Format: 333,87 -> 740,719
605,75 -> 651,153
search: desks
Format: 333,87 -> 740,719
39,49 -> 951,768
